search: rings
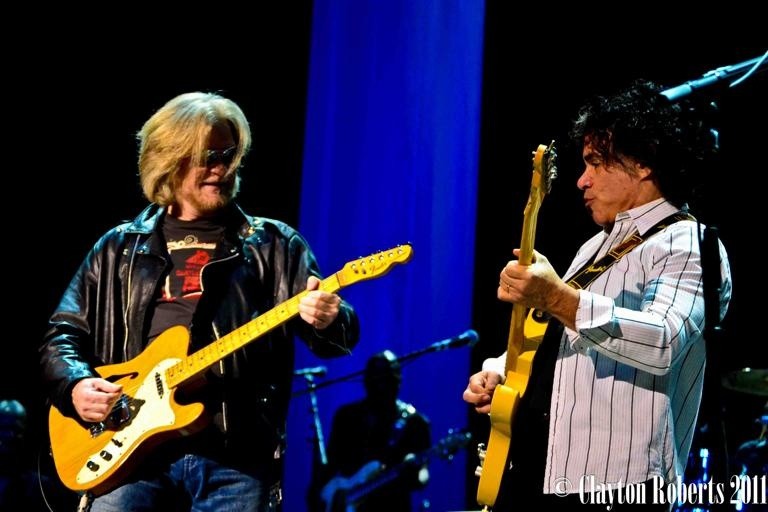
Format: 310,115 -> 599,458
506,285 -> 511,294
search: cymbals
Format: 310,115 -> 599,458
721,367 -> 767,395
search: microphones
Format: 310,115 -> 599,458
430,329 -> 477,352
294,365 -> 328,378
655,52 -> 768,105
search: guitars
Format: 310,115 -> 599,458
475,138 -> 558,511
48,241 -> 414,497
320,429 -> 473,512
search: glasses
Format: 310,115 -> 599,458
198,145 -> 238,169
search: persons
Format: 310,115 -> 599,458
461,79 -> 738,508
37,91 -> 359,510
309,346 -> 432,510
